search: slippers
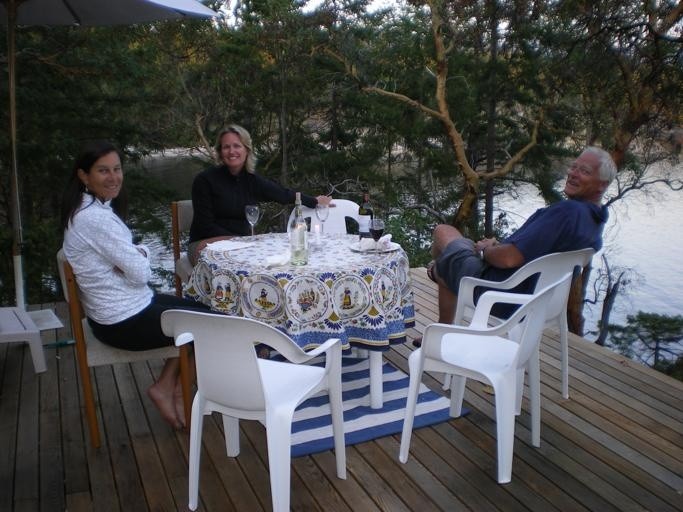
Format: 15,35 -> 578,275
427,260 -> 440,283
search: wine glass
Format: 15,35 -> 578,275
244,205 -> 260,242
368,219 -> 384,262
315,204 -> 329,239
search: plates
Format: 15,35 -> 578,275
350,242 -> 401,253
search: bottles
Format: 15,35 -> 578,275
359,195 -> 373,240
290,192 -> 308,266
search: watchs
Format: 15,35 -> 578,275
478,244 -> 489,262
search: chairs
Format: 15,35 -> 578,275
160,307 -> 349,512
169,199 -> 194,298
397,269 -> 577,484
285,198 -> 363,235
58,248 -> 193,451
439,245 -> 598,420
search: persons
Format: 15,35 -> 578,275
60,141 -> 231,430
412,146 -> 617,347
187,124 -> 337,268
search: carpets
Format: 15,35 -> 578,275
260,350 -> 473,459
